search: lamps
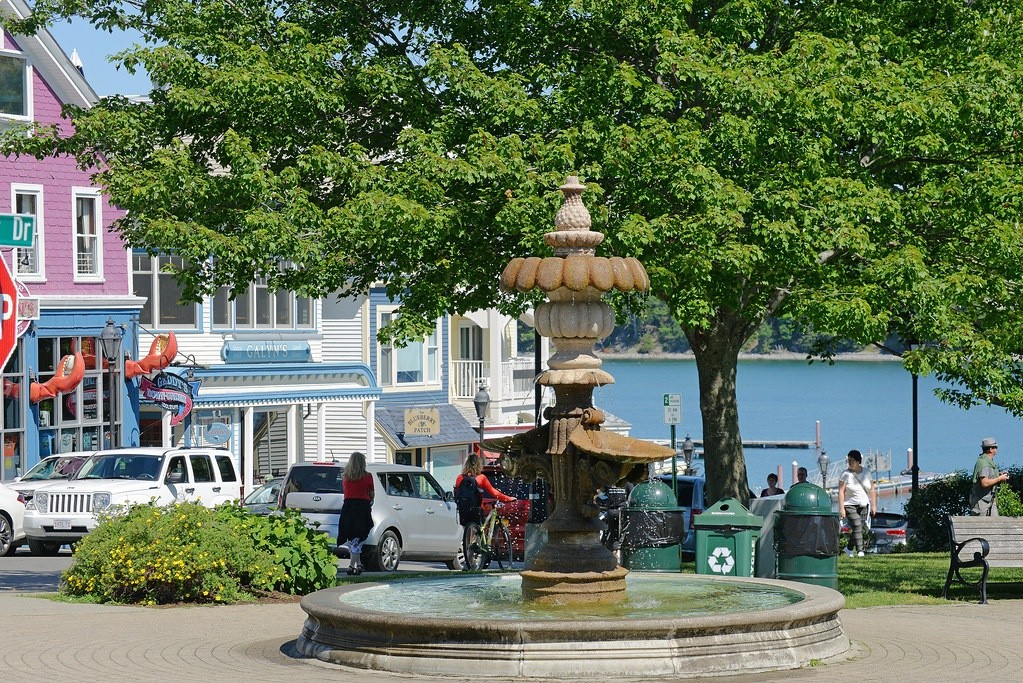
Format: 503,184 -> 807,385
1,247 -> 29,265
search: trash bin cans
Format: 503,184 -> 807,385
621,479 -> 685,572
692,494 -> 764,576
774,481 -> 842,591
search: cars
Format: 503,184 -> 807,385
4,450 -> 100,503
239,476 -> 285,516
0,482 -> 27,557
841,510 -> 908,554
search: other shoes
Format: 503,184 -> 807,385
462,562 -> 479,571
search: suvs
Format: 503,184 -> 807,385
23,445 -> 244,557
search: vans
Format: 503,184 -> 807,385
275,461 -> 485,572
479,466 -> 530,561
653,476 -> 706,552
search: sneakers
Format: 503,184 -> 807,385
844,547 -> 855,557
857,551 -> 865,557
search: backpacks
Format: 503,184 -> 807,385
456,474 -> 482,511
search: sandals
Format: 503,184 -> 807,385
347,566 -> 355,575
356,565 -> 362,575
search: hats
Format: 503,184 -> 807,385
981,438 -> 998,447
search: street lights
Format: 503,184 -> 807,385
816,447 -> 830,492
96,315 -> 123,448
682,434 -> 695,476
473,383 -> 491,464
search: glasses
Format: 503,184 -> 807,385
990,446 -> 997,449
846,459 -> 855,463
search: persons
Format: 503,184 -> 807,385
388,476 -> 414,496
970,438 -> 1010,516
760,474 -> 785,497
156,460 -> 172,479
790,467 -> 810,488
457,452 -> 517,570
838,450 -> 876,557
337,452 -> 375,575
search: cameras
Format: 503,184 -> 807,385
1003,473 -> 1009,477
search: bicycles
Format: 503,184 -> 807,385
462,498 -> 518,572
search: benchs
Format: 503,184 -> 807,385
941,514 -> 1023,605
